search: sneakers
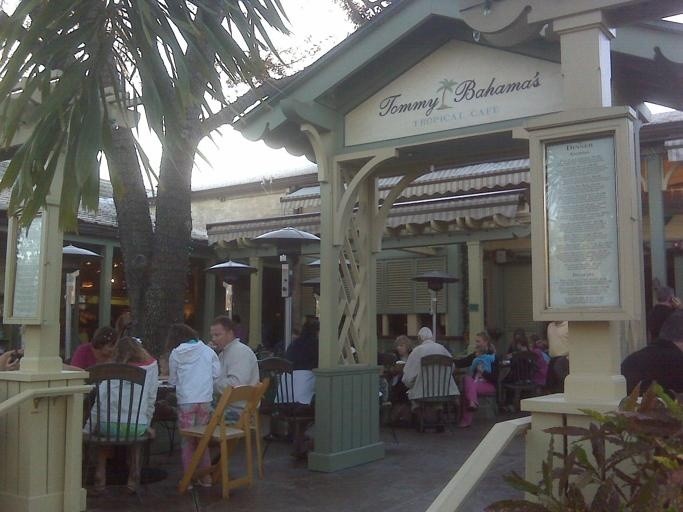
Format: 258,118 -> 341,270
194,475 -> 212,487
467,400 -> 480,410
177,479 -> 193,490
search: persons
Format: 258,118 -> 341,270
0,349 -> 85,371
267,312 -> 320,459
620,310 -> 682,404
73,311 -> 261,498
648,286 -> 682,341
379,327 -> 552,434
